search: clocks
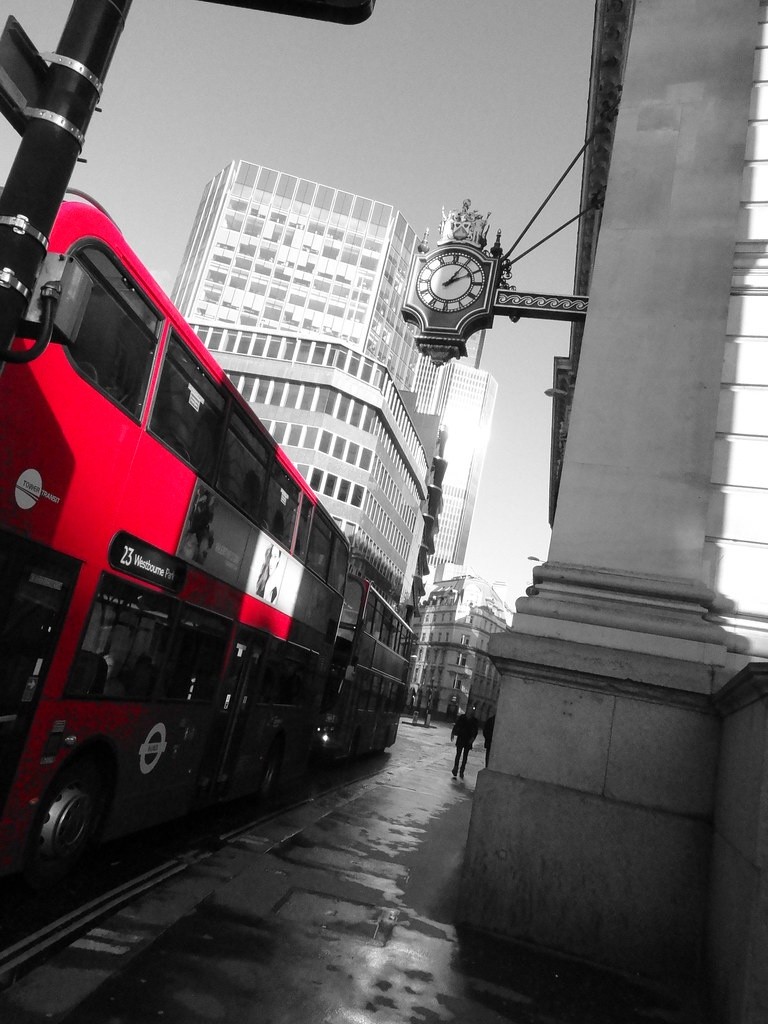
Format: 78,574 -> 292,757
416,252 -> 486,313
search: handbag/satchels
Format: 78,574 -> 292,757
454,720 -> 463,736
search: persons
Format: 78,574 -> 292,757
451,705 -> 478,778
188,487 -> 217,557
257,544 -> 281,603
482,715 -> 495,768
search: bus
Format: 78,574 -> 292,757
0,198 -> 353,888
303,572 -> 420,770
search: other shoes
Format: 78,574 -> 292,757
459,773 -> 465,779
452,770 -> 458,777
